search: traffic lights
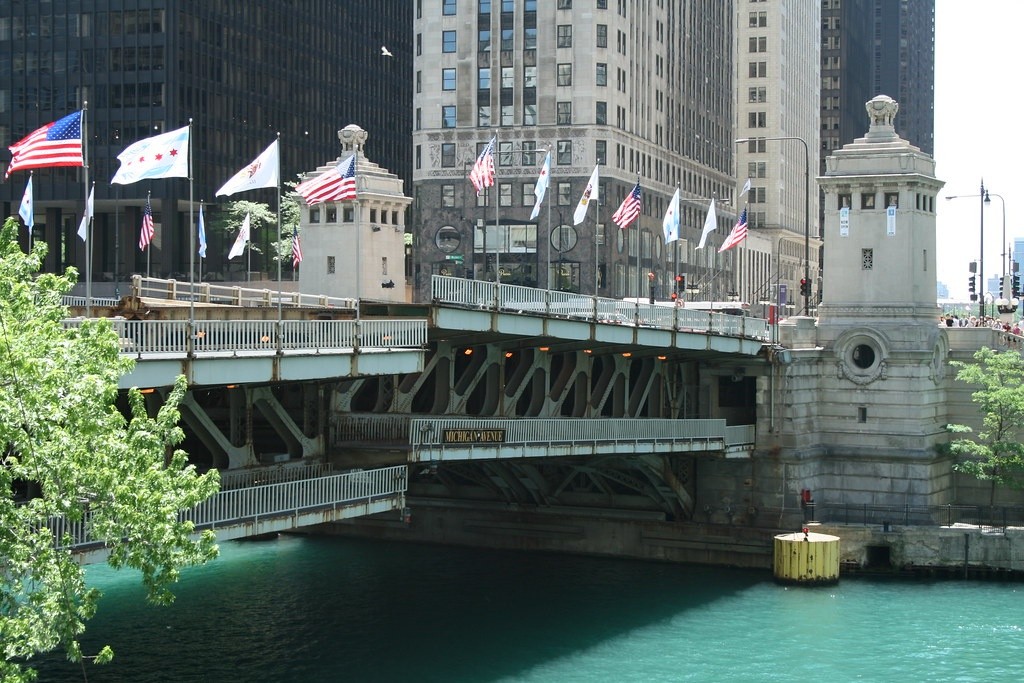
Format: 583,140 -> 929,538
999,277 -> 1004,296
969,276 -> 975,293
800,278 -> 807,296
1014,276 -> 1021,296
809,278 -> 812,296
800,488 -> 815,522
675,275 -> 685,292
672,293 -> 676,301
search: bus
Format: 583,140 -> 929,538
618,297 -> 751,335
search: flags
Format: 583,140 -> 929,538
292,225 -> 303,269
295,154 -> 357,208
77,187 -> 94,242
573,164 -> 599,226
199,205 -> 208,258
139,198 -> 155,252
663,187 -> 679,245
529,152 -> 550,220
612,177 -> 642,230
717,206 -> 748,253
694,198 -> 717,249
214,139 -> 278,198
739,178 -> 751,198
469,135 -> 497,192
227,213 -> 250,260
5,110 -> 83,179
111,127 -> 189,185
19,175 -> 35,234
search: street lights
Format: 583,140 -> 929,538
483,149 -> 546,281
675,198 -> 730,305
986,291 -> 994,318
735,137 -> 810,317
945,194 -> 1006,278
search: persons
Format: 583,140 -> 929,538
940,313 -> 1024,349
